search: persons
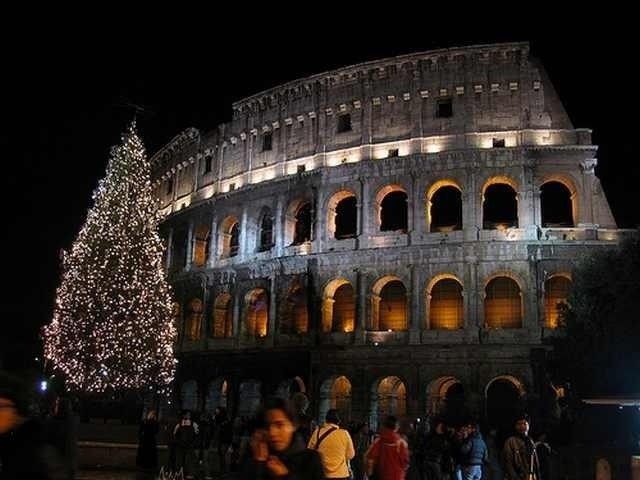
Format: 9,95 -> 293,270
0,396 -> 44,479
532,425 -> 546,480
308,408 -> 489,480
484,433 -> 504,480
138,407 -> 243,480
236,397 -> 326,480
501,412 -> 541,480
